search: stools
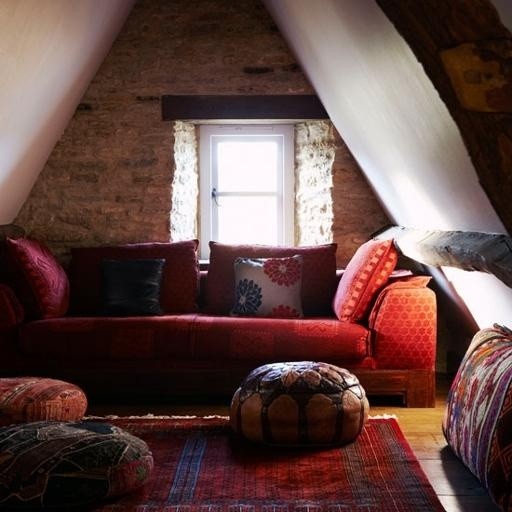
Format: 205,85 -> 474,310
229,361 -> 369,450
0,376 -> 88,429
0,418 -> 153,508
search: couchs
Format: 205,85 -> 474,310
0,224 -> 437,408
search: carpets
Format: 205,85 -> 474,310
84,414 -> 446,512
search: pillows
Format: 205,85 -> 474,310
230,254 -> 303,319
86,238 -> 199,315
333,237 -> 398,324
440,323 -> 512,512
207,240 -> 336,315
100,258 -> 164,317
5,237 -> 70,320
